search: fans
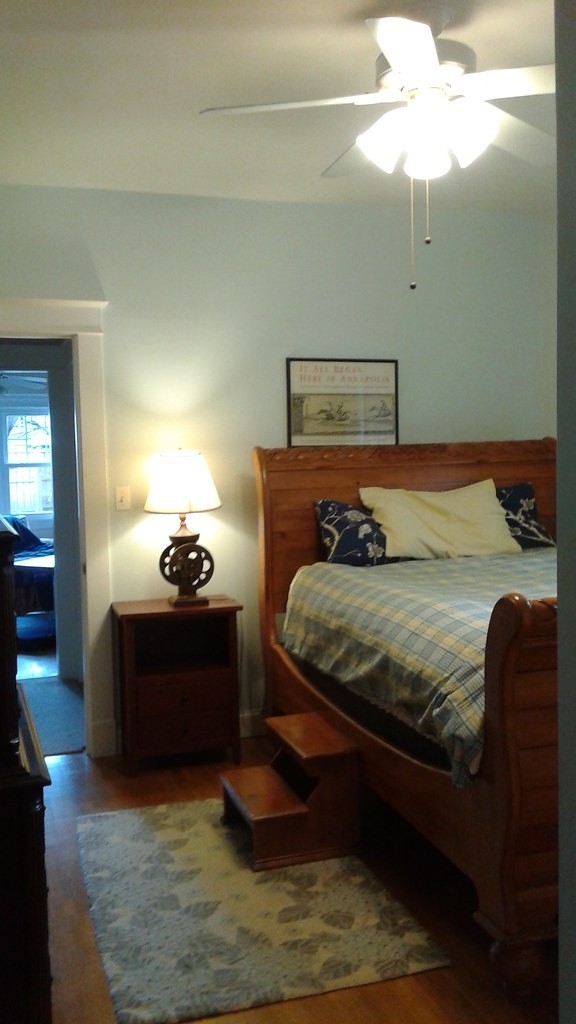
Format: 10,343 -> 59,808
200,17 -> 556,182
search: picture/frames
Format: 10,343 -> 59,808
286,356 -> 400,448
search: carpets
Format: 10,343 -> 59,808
74,796 -> 450,1024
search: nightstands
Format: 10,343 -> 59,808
110,593 -> 245,776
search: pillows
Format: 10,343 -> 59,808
357,478 -> 524,559
496,482 -> 558,550
315,498 -> 410,567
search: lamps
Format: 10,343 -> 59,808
355,90 -> 490,290
143,450 -> 224,608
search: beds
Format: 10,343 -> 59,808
252,436 -> 559,1008
